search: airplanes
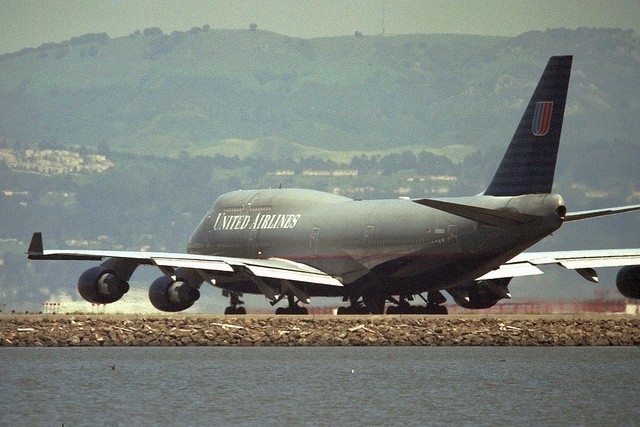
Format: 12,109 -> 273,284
25,55 -> 640,314
